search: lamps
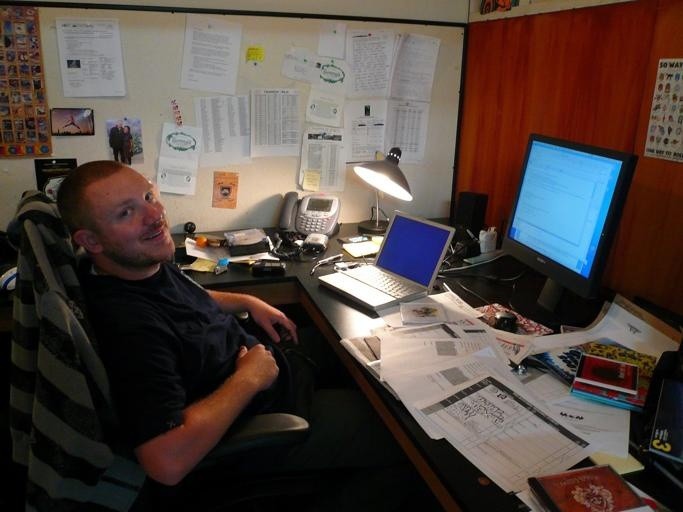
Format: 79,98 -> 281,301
353,146 -> 414,235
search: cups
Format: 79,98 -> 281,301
480,230 -> 497,252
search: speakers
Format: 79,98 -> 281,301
454,189 -> 492,244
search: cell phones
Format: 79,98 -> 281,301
252,262 -> 287,276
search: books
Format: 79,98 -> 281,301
527,465 -> 653,512
569,344 -> 658,411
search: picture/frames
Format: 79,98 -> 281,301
34,158 -> 77,192
50,107 -> 95,136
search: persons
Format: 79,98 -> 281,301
122,126 -> 133,159
58,161 -> 379,485
110,123 -> 123,162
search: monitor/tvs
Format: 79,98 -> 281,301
494,126 -> 638,326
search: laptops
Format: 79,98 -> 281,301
317,208 -> 457,315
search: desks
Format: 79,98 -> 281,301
175,218 -> 683,511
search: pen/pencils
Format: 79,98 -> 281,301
466,229 -> 479,242
513,343 -> 525,375
441,324 -> 460,338
317,254 -> 344,264
443,283 -> 452,292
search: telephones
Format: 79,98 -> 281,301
272,192 -> 340,258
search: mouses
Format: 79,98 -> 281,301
494,308 -> 519,328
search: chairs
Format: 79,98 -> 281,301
7,191 -> 308,464
